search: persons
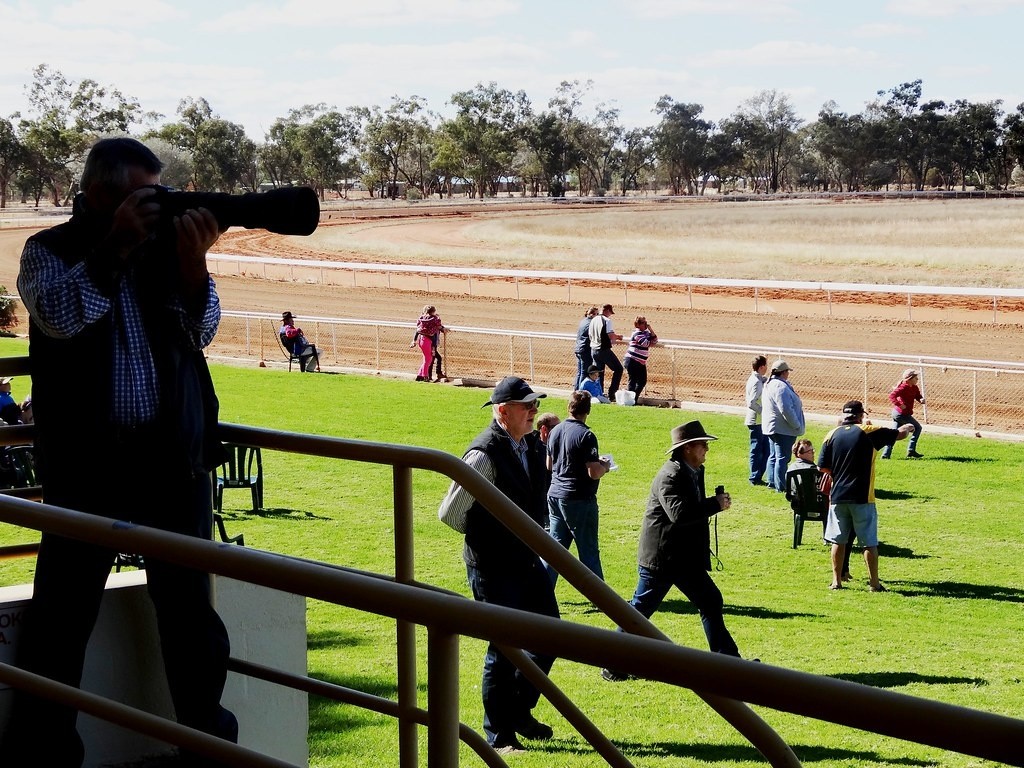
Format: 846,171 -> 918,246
817,402 -> 915,595
745,356 -> 769,487
601,422 -> 760,680
438,375 -> 562,755
279,312 -> 324,373
789,439 -> 831,511
623,316 -> 658,405
573,306 -> 600,391
10,138 -> 238,768
589,305 -> 624,402
0,377 -> 35,425
881,368 -> 925,460
535,413 -> 562,490
761,360 -> 805,492
410,305 -> 451,383
579,364 -> 610,404
538,388 -> 612,605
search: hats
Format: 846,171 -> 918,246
603,304 -> 615,314
903,368 -> 918,381
843,400 -> 869,415
0,377 -> 13,384
664,420 -> 719,456
771,360 -> 793,373
280,312 -> 297,322
481,376 -> 547,409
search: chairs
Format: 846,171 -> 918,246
0,446 -> 41,502
280,334 -> 321,373
215,514 -> 245,545
785,468 -> 829,550
210,442 -> 263,514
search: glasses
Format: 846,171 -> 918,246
690,442 -> 708,447
641,322 -> 647,324
801,449 -> 815,454
591,313 -> 599,315
539,425 -> 556,431
502,400 -> 540,410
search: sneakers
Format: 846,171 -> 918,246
493,739 -> 528,759
513,707 -> 554,740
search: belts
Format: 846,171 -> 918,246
112,421 -> 180,445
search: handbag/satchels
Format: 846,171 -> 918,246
293,332 -> 309,355
614,389 -> 636,406
818,471 -> 832,495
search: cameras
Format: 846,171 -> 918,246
138,184 -> 321,236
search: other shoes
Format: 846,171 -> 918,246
416,371 -> 446,382
751,479 -> 793,493
829,571 -> 886,595
908,451 -> 924,458
882,455 -> 890,460
602,666 -> 629,682
